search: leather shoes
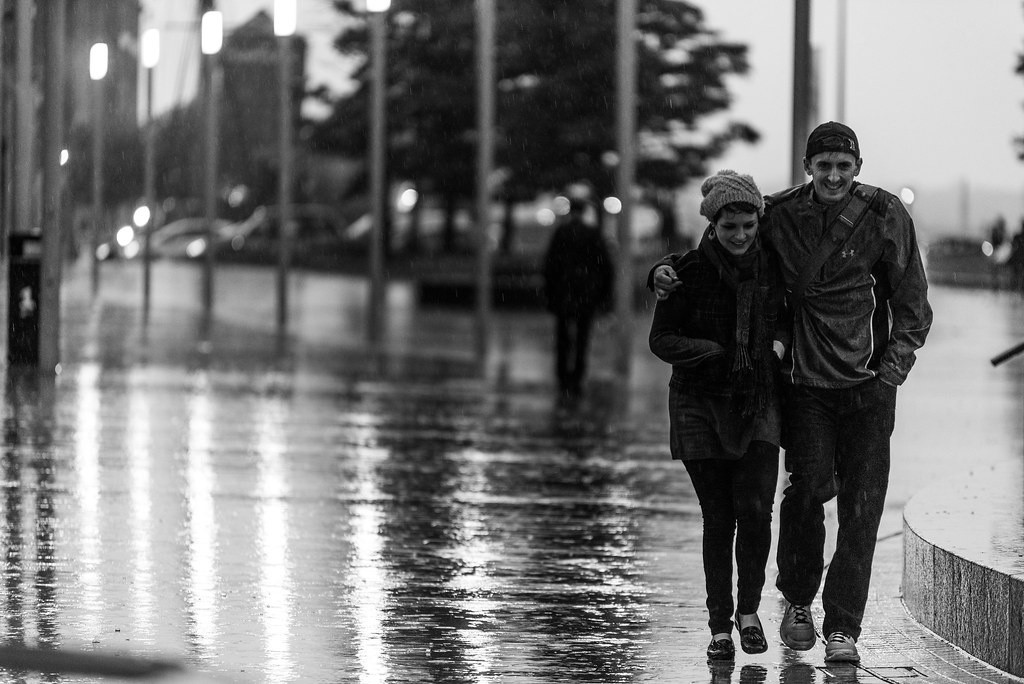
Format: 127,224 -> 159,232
735,609 -> 769,654
707,638 -> 735,660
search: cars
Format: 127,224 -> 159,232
192,204 -> 350,269
116,218 -> 230,263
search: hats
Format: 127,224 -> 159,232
699,169 -> 763,223
805,121 -> 860,161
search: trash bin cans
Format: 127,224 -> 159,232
6,227 -> 43,362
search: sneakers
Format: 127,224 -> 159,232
824,632 -> 861,662
779,600 -> 816,651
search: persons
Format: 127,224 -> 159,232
984,228 -> 1024,291
536,197 -> 616,413
646,121 -> 933,662
649,170 -> 790,659
